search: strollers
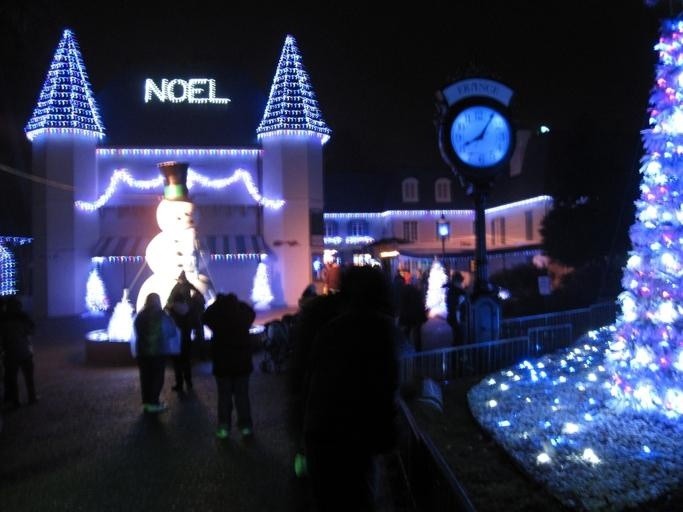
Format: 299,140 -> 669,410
259,320 -> 300,376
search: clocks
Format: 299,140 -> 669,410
448,106 -> 517,172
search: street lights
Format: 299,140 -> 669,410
436,210 -> 451,264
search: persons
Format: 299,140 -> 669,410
0,292 -> 43,408
135,281 -> 256,440
445,271 -> 468,347
258,264 -> 428,510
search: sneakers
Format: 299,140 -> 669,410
172,385 -> 183,390
215,428 -> 230,440
239,428 -> 254,441
142,401 -> 166,414
